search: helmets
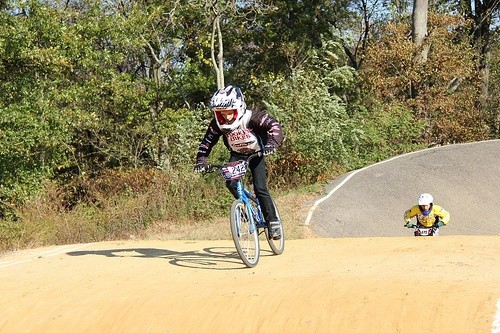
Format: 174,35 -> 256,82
208,84 -> 248,129
417,193 -> 434,215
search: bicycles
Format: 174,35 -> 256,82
193,146 -> 285,268
404,224 -> 447,236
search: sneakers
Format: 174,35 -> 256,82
239,208 -> 248,222
268,221 -> 280,238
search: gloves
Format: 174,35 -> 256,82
407,223 -> 413,227
262,144 -> 274,156
437,221 -> 443,226
194,161 -> 205,173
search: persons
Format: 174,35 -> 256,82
403,193 -> 450,236
194,84 -> 283,239
